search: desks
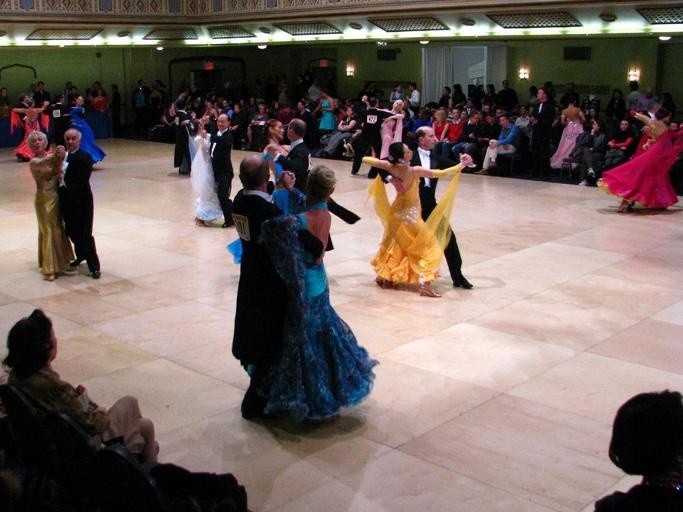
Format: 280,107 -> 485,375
0,110 -> 112,150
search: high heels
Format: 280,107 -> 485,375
420,283 -> 442,297
615,204 -> 629,213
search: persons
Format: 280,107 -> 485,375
351,78 -> 683,195
2,80 -> 120,166
232,155 -> 325,426
131,78 -> 363,161
594,390 -> 682,512
27,128 -> 100,280
228,117 -> 310,263
261,166 -> 378,426
3,310 -> 159,462
363,126 -> 474,296
181,112 -> 234,228
174,99 -> 214,175
599,103 -> 683,212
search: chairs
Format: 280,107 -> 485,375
68,447 -> 169,510
0,379 -> 64,512
156,93 -> 683,183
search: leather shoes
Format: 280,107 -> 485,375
241,410 -> 290,420
92,270 -> 100,278
473,169 -> 490,175
222,222 -> 235,228
454,278 -> 473,289
70,258 -> 83,266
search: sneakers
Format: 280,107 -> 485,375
587,167 -> 596,178
577,179 -> 588,186
